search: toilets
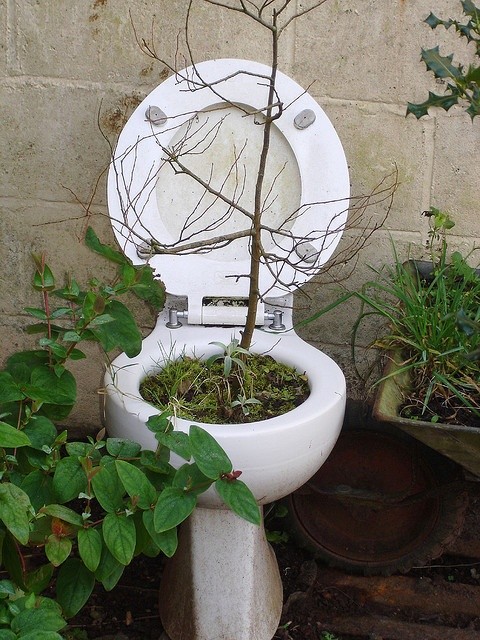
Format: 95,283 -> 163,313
101,57 -> 351,640
